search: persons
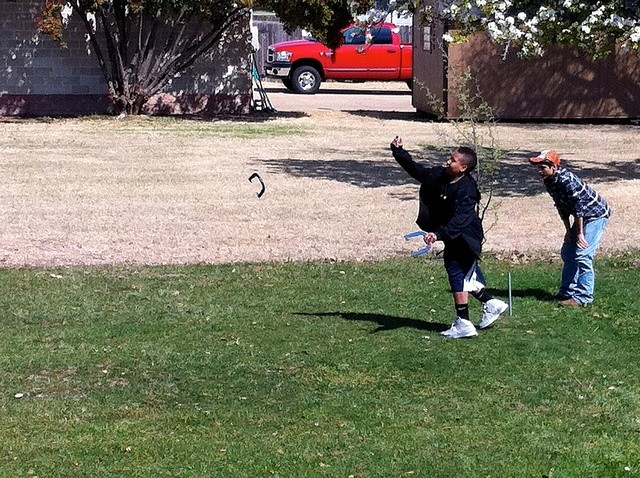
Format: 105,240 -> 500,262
528,150 -> 612,309
391,134 -> 510,339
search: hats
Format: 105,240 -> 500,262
529,148 -> 561,167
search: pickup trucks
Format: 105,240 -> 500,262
263,21 -> 413,94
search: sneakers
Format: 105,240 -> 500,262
479,300 -> 510,330
559,299 -> 583,308
555,295 -> 568,302
440,317 -> 480,340
